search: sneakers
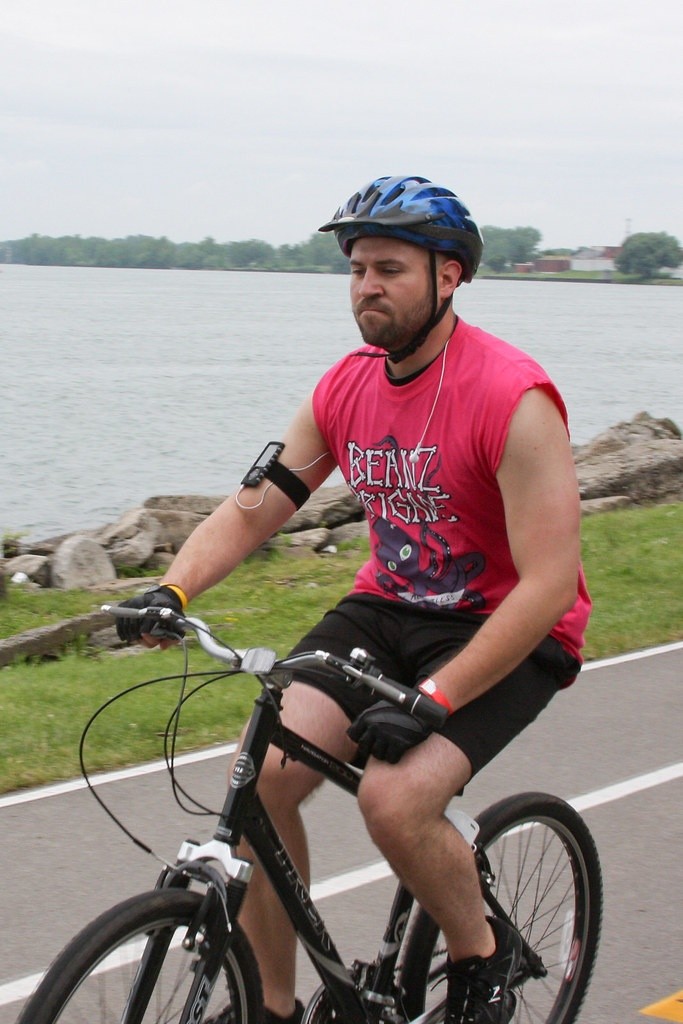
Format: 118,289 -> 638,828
432,915 -> 524,1024
203,995 -> 304,1023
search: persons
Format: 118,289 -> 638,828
113,168 -> 596,1024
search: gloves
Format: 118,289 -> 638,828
346,688 -> 432,765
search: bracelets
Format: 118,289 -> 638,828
418,678 -> 455,719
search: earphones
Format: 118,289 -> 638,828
409,442 -> 421,463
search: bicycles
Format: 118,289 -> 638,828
13,601 -> 604,1024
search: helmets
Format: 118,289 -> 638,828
317,175 -> 484,284
